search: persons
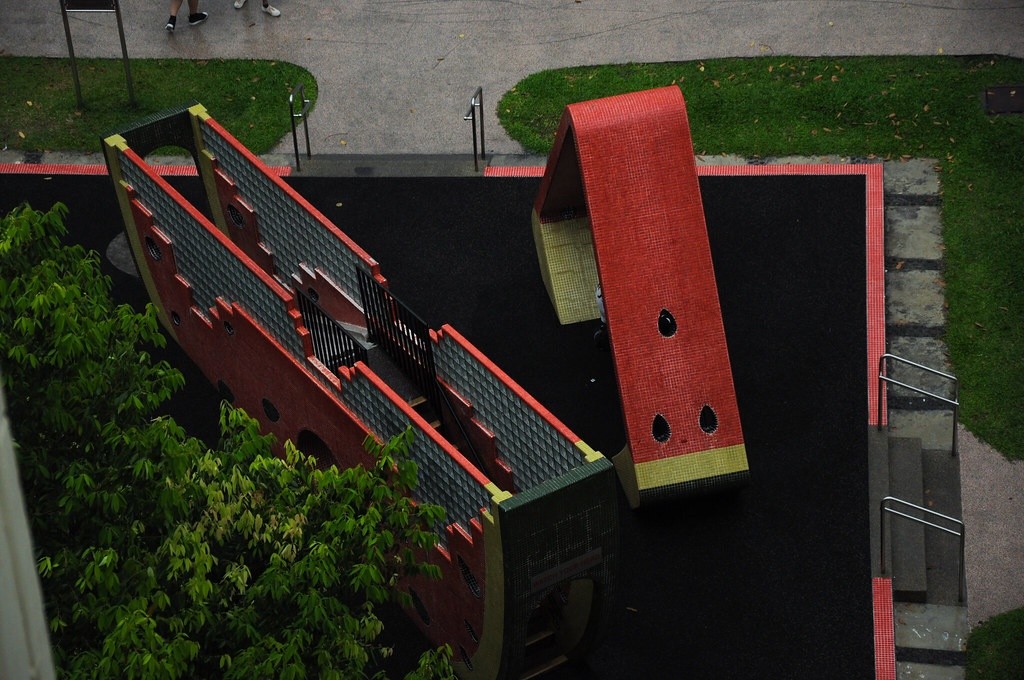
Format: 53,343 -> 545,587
165,0 -> 208,30
234,0 -> 281,17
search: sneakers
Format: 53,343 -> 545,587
189,12 -> 208,25
234,0 -> 245,8
262,4 -> 280,16
165,16 -> 176,32
